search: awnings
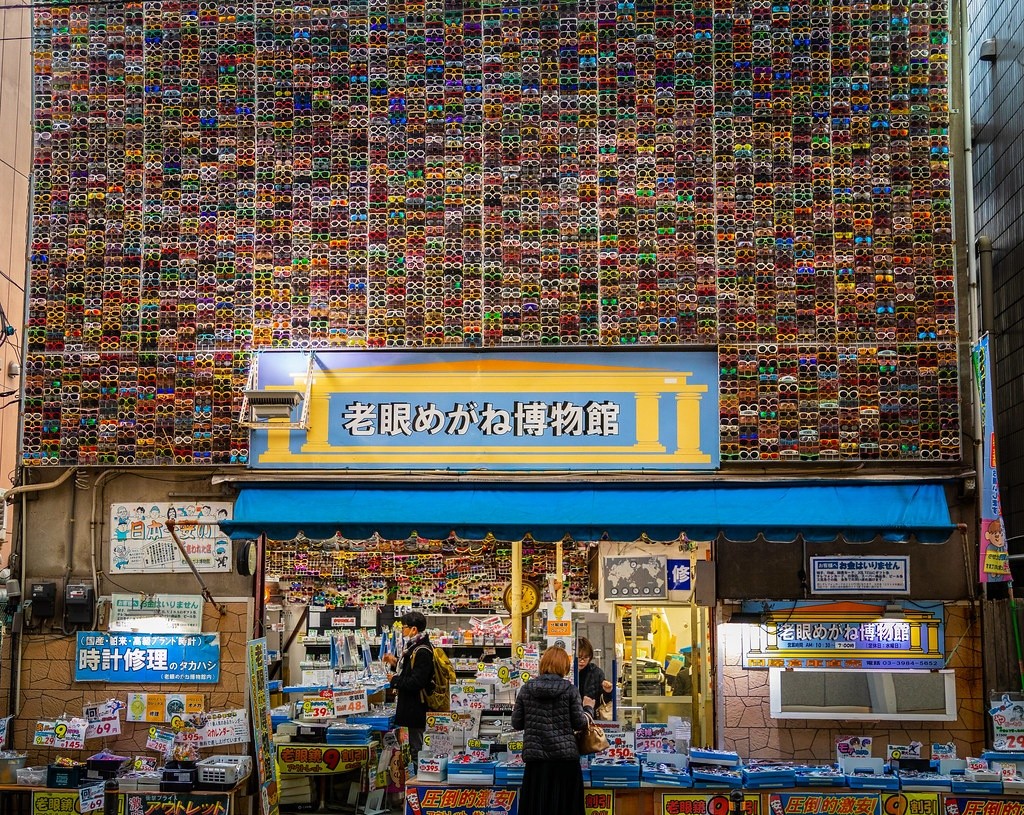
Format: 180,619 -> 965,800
167,484 -> 980,617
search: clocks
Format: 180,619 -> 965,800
503,580 -> 539,616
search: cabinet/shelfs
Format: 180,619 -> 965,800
300,609 -> 531,683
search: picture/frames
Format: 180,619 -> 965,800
603,555 -> 668,600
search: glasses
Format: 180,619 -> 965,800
661,542 -> 674,546
402,626 -> 411,630
21,0 -> 961,465
265,539 -> 589,616
642,539 -> 657,544
579,656 -> 590,661
676,538 -> 685,542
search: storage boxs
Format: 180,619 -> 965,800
86,753 -> 131,781
196,755 -> 252,783
271,715 -> 292,731
16,766 -> 46,787
277,722 -> 297,736
279,776 -> 313,804
269,680 -> 283,690
46,762 -> 87,789
273,733 -> 290,744
267,650 -> 280,660
297,605 -> 1023,796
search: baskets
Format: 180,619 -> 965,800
195,754 -> 253,783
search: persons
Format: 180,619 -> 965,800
512,645 -> 596,815
381,612 -> 436,780
672,648 -> 701,695
572,636 -> 612,721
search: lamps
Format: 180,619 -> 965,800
238,351 -> 314,431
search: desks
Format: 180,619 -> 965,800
404,775 -> 1024,815
0,786 -> 246,815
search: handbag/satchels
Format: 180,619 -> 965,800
576,712 -> 610,756
596,694 -> 613,720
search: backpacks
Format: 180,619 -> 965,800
409,642 -> 456,712
